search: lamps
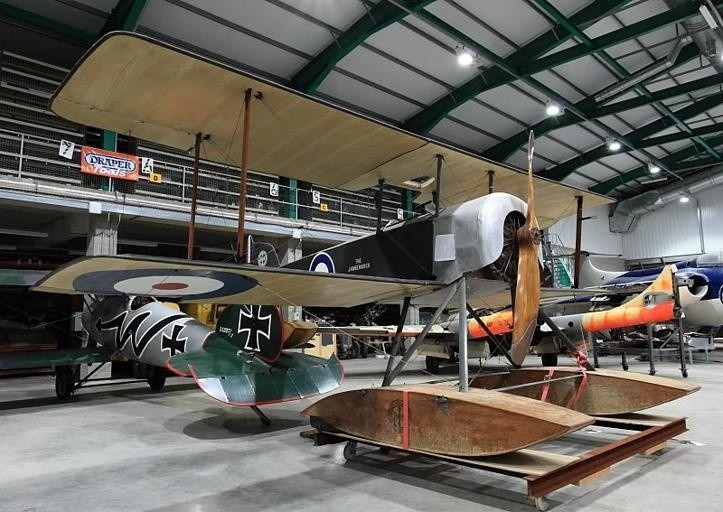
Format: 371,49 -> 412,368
452,40 -> 692,206
